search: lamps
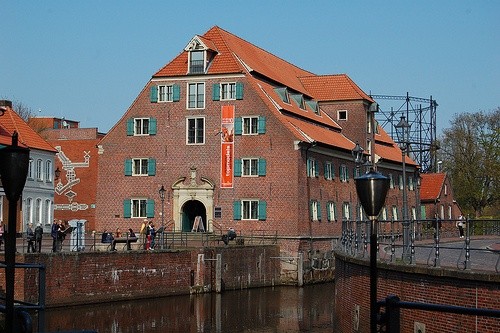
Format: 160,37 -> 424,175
54,167 -> 61,180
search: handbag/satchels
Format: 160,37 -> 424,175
27,239 -> 31,242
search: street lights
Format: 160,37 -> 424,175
353,164 -> 391,333
351,143 -> 365,257
437,160 -> 444,175
393,112 -> 412,263
0,131 -> 31,333
158,185 -> 167,250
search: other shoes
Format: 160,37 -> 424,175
151,249 -> 154,250
149,247 -> 151,249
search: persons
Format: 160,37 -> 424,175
456,212 -> 466,238
27,223 -> 44,253
139,219 -> 156,250
431,213 -> 443,241
0,221 -> 4,252
51,219 -> 66,252
128,228 -> 136,237
102,227 -> 122,240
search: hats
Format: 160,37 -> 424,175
37,223 -> 40,226
144,219 -> 148,221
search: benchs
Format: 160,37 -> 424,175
92,243 -> 141,251
